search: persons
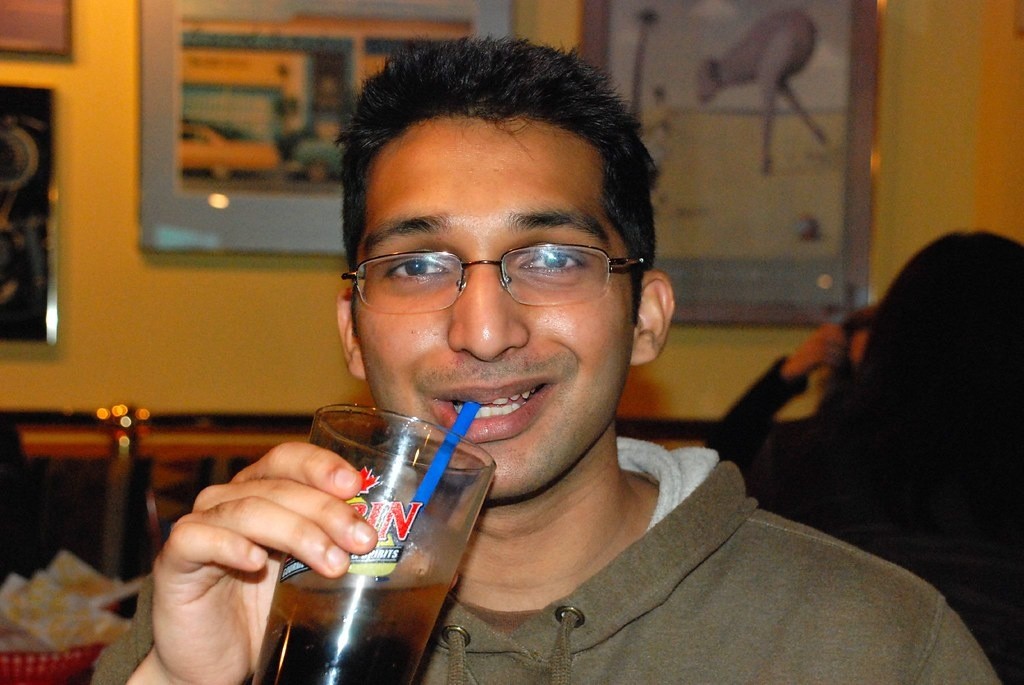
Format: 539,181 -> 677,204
89,34 -> 1004,685
706,228 -> 1023,685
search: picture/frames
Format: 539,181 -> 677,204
137,1 -> 510,261
579,0 -> 884,325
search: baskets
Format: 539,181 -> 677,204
0,602 -> 117,685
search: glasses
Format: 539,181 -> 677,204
341,243 -> 644,314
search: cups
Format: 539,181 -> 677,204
252,403 -> 497,685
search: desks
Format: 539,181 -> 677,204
20,424 -> 311,617
